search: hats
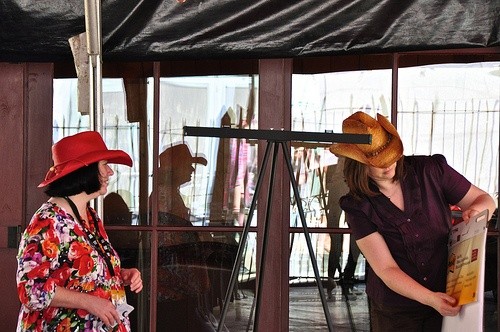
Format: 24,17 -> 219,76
151,144 -> 208,172
36,131 -> 133,190
329,112 -> 402,168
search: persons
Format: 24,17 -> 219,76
101,144 -> 359,332
329,111 -> 496,332
15,131 -> 143,332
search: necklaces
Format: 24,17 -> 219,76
387,188 -> 397,200
